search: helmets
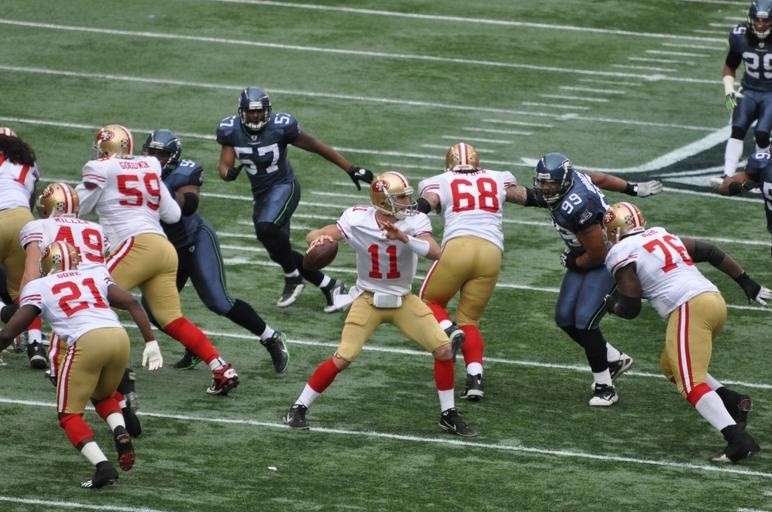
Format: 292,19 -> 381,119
92,125 -> 134,158
369,171 -> 418,220
36,183 -> 80,218
445,142 -> 480,172
237,85 -> 274,132
142,129 -> 182,180
602,202 -> 645,250
746,0 -> 771,42
39,241 -> 79,278
534,152 -> 574,207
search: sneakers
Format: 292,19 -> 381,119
206,362 -> 239,395
592,350 -> 634,392
174,349 -> 202,371
114,366 -> 142,471
589,383 -> 619,407
438,408 -> 478,437
276,273 -> 305,308
282,402 -> 310,431
13,332 -> 49,370
80,461 -> 120,490
459,374 -> 485,401
444,321 -> 465,362
712,391 -> 762,464
260,330 -> 290,374
319,279 -> 346,314
710,176 -> 726,187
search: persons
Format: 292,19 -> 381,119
598,202 -> 772,465
140,129 -> 291,375
280,170 -> 478,438
0,124 -> 49,370
0,246 -> 163,487
215,86 -> 373,313
72,124 -> 240,397
536,152 -> 662,408
407,141 -> 547,399
17,182 -> 142,439
721,133 -> 772,232
722,2 -> 772,178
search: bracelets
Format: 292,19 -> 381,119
406,235 -> 429,258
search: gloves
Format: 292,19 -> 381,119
605,292 -> 616,313
725,88 -> 744,111
142,340 -> 163,372
347,167 -> 374,190
225,162 -> 245,181
630,180 -> 664,197
738,274 -> 772,307
560,251 -> 571,267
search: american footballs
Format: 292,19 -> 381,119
303,234 -> 338,271
